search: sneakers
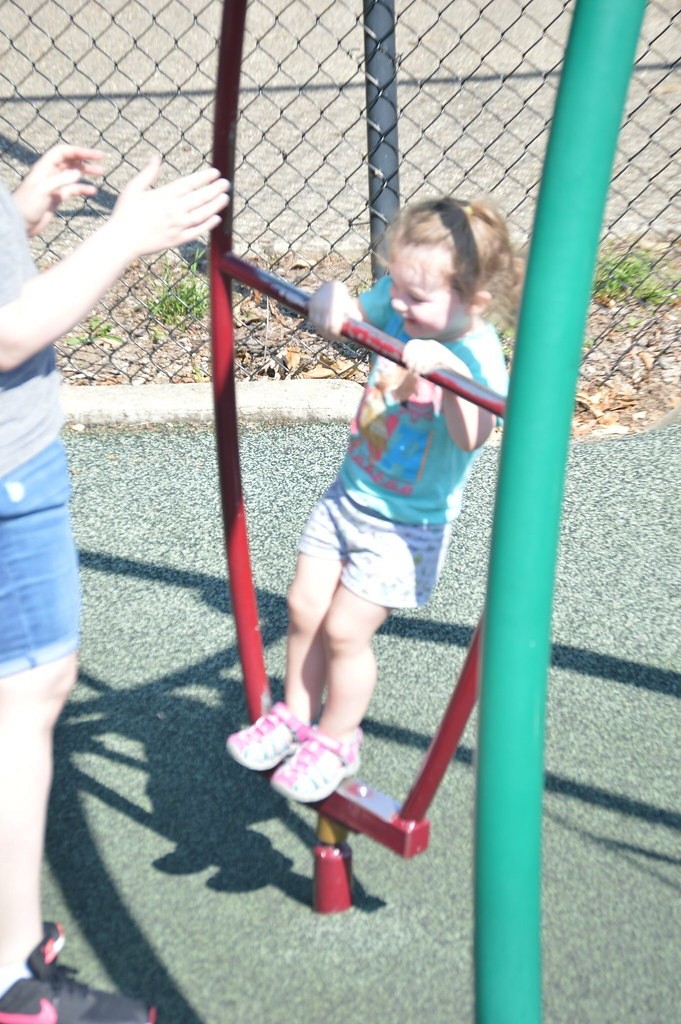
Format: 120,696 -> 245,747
270,720 -> 363,803
0,921 -> 157,1024
226,701 -> 312,771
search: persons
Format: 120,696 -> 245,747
225,195 -> 511,804
0,141 -> 229,1024
